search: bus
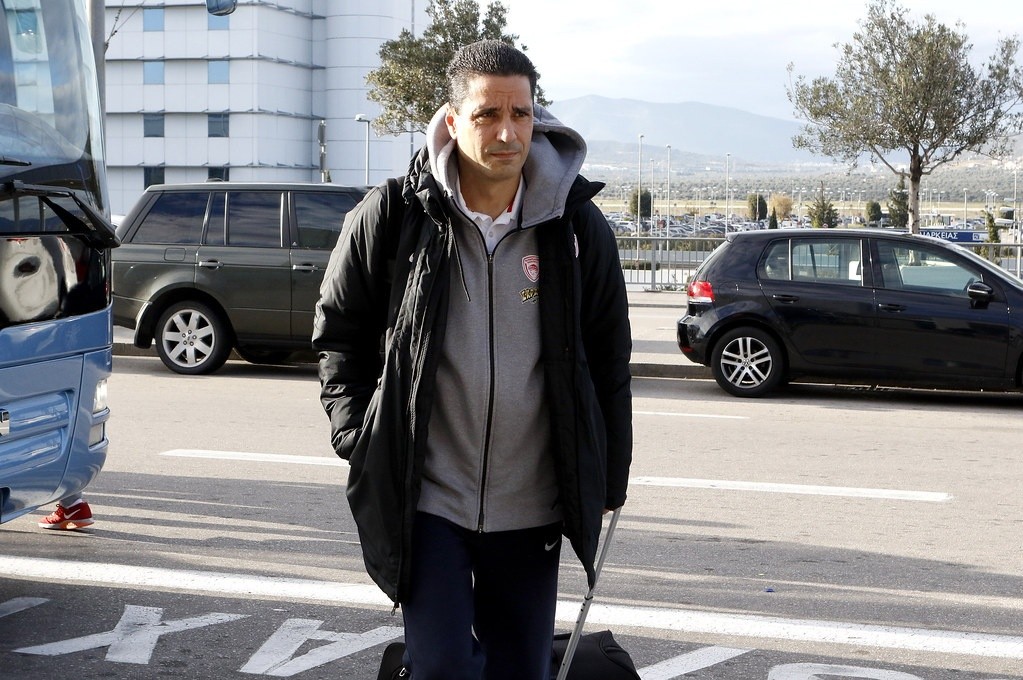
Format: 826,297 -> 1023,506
0,0 -> 124,516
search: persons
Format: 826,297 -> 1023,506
37,492 -> 96,530
309,38 -> 633,680
53,252 -> 107,319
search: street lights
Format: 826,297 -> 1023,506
636,135 -> 641,261
354,113 -> 371,186
666,145 -> 671,253
963,188 -> 968,230
650,159 -> 654,237
1013,171 -> 1017,244
724,153 -> 731,236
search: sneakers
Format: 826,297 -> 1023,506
38,502 -> 94,529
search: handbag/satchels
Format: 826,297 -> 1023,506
377,627 -> 642,680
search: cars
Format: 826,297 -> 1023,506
603,211 -> 1016,238
674,229 -> 1023,397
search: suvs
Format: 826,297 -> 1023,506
104,181 -> 383,375
